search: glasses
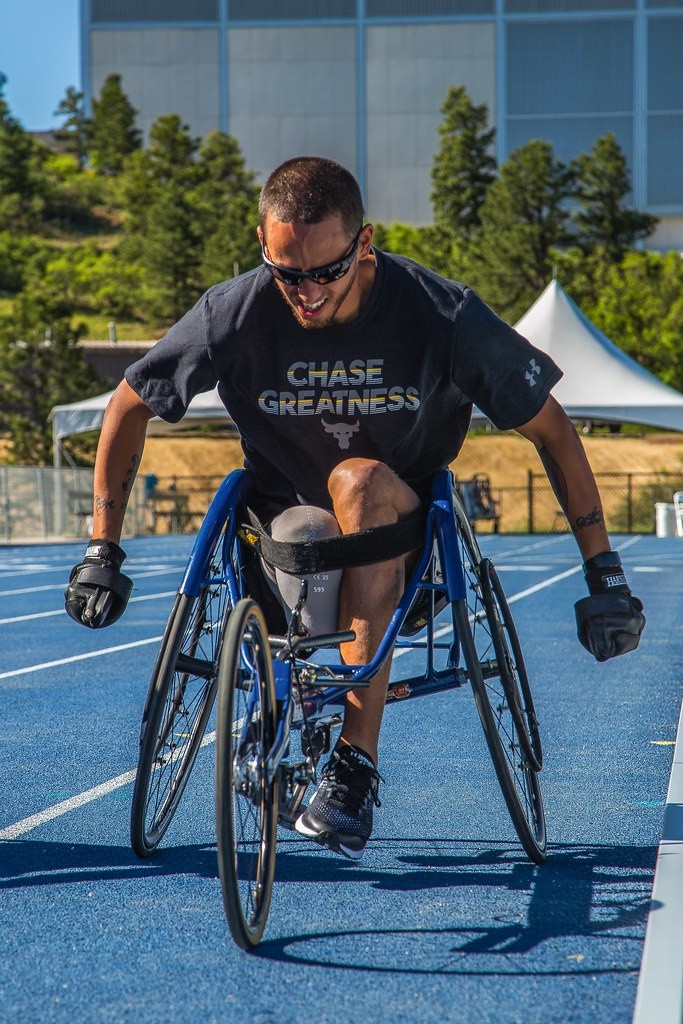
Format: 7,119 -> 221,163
261,217 -> 363,286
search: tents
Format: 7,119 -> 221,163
50,380 -> 231,535
472,278 -> 683,430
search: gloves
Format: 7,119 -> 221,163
64,539 -> 133,629
574,551 -> 646,662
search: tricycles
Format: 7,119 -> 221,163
130,469 -> 549,951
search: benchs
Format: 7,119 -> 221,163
67,490 -> 94,537
150,495 -> 205,533
453,478 -> 501,534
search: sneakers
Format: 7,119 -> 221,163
294,745 -> 386,860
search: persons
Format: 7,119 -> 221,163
66,156 -> 646,857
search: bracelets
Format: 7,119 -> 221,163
582,551 -> 621,574
